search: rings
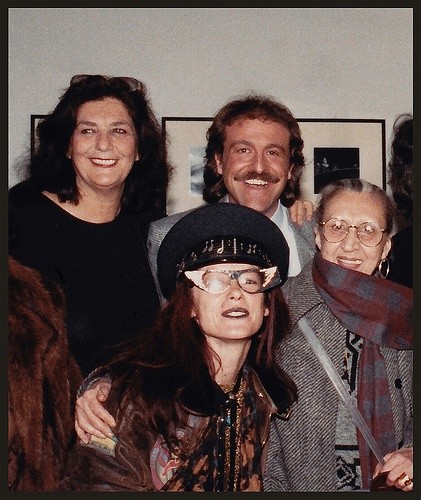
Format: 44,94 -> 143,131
398,471 -> 412,486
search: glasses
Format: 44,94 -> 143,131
316,218 -> 389,246
69,74 -> 142,91
175,267 -> 281,294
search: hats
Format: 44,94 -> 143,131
156,204 -> 290,297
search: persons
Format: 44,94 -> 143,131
316,157 -> 329,174
57,203 -> 300,492
9,74 -> 317,379
75,178 -> 414,493
146,89 -> 317,311
378,113 -> 413,289
8,258 -> 89,492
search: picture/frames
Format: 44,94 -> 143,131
31,115 -> 49,160
162,116 -> 215,217
295,119 -> 386,206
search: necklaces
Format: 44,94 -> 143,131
218,372 -> 237,393
